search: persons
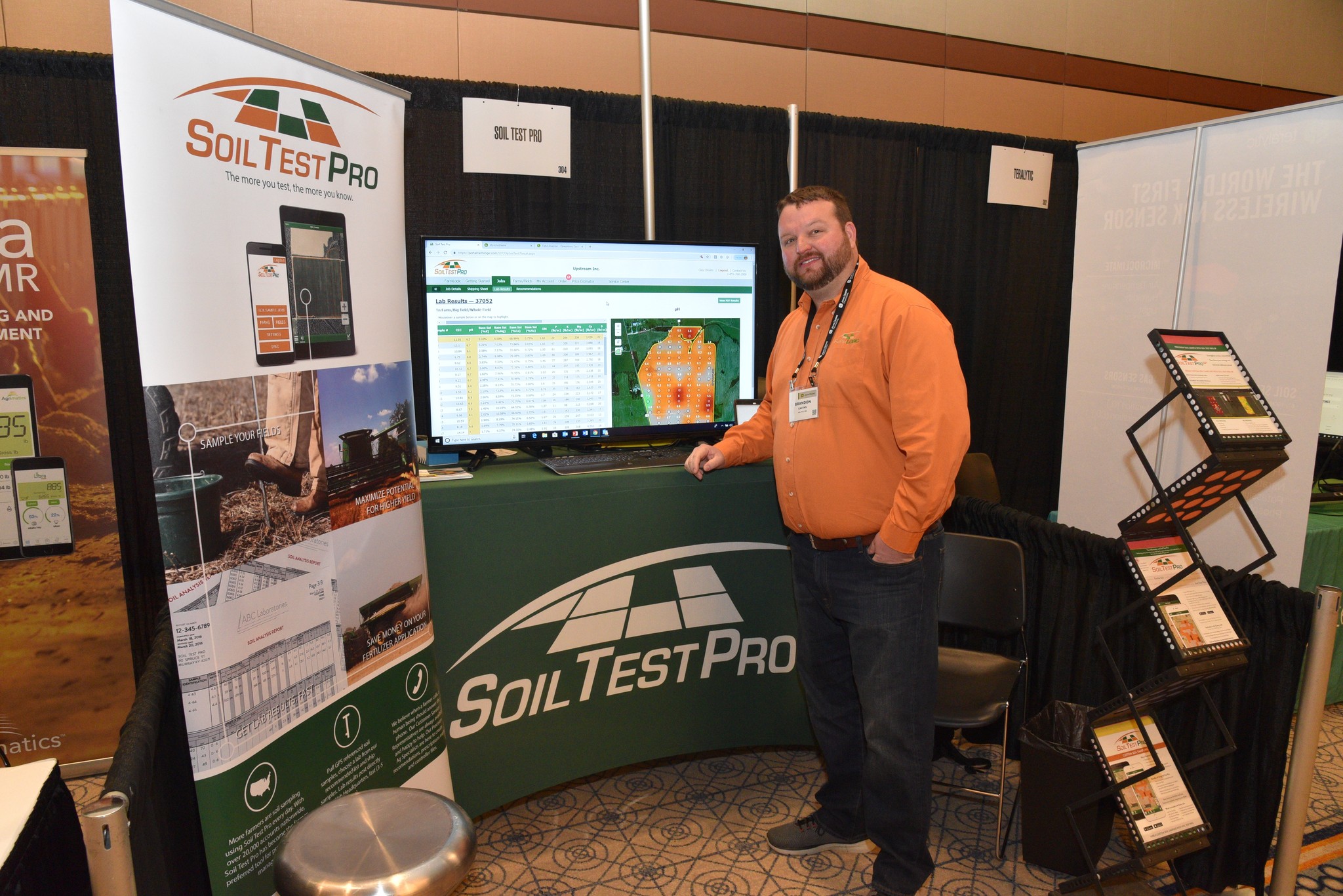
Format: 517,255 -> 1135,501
683,183 -> 972,896
245,369 -> 328,516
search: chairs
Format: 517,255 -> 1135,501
924,532 -> 1035,860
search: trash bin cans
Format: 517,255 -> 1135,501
1020,699 -> 1116,876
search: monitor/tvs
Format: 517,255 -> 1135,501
420,233 -> 762,444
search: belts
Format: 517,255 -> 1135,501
801,518 -> 941,552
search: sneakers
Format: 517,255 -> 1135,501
765,807 -> 876,856
245,453 -> 303,496
289,490 -> 328,516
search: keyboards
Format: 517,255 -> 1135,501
539,449 -> 692,475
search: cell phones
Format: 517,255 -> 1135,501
242,242 -> 296,367
10,455 -> 75,558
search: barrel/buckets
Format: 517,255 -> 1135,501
153,469 -> 227,573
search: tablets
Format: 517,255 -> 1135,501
0,373 -> 40,563
277,204 -> 357,361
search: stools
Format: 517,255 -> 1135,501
275,787 -> 480,895
